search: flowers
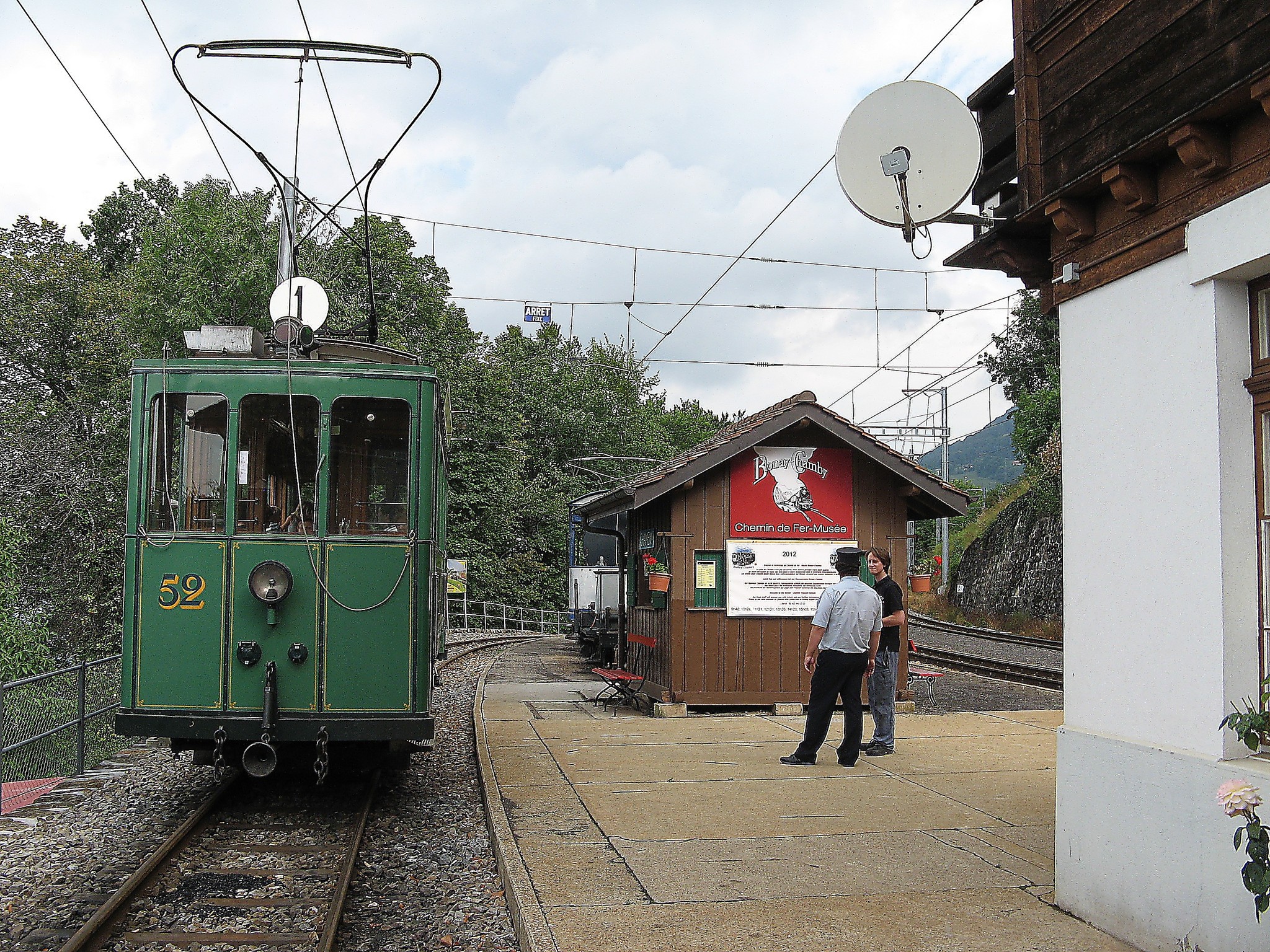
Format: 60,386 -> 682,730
1217,777 -> 1270,924
906,555 -> 942,576
641,553 -> 667,574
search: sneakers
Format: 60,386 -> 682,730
860,739 -> 877,751
838,758 -> 855,767
780,754 -> 817,765
866,742 -> 896,757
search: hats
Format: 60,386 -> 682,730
837,547 -> 861,563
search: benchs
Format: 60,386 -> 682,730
591,633 -> 657,719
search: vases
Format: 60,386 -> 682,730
907,574 -> 931,592
647,571 -> 673,592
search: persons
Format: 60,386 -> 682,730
280,501 -> 313,531
265,506 -> 282,528
237,498 -> 248,528
377,500 -> 404,532
780,547 -> 883,768
266,505 -> 273,521
860,547 -> 905,756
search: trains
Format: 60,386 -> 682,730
113,37 -> 448,795
